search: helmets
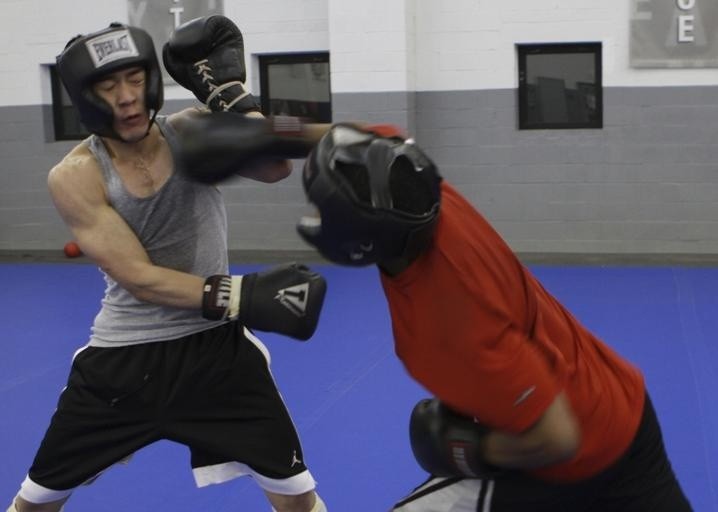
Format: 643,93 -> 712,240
56,23 -> 164,145
296,121 -> 440,267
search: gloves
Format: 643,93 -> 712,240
202,261 -> 327,341
409,397 -> 494,480
162,15 -> 262,114
167,111 -> 308,186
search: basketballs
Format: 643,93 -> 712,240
64,241 -> 80,257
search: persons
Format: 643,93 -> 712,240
164,15 -> 694,512
1,21 -> 406,510
